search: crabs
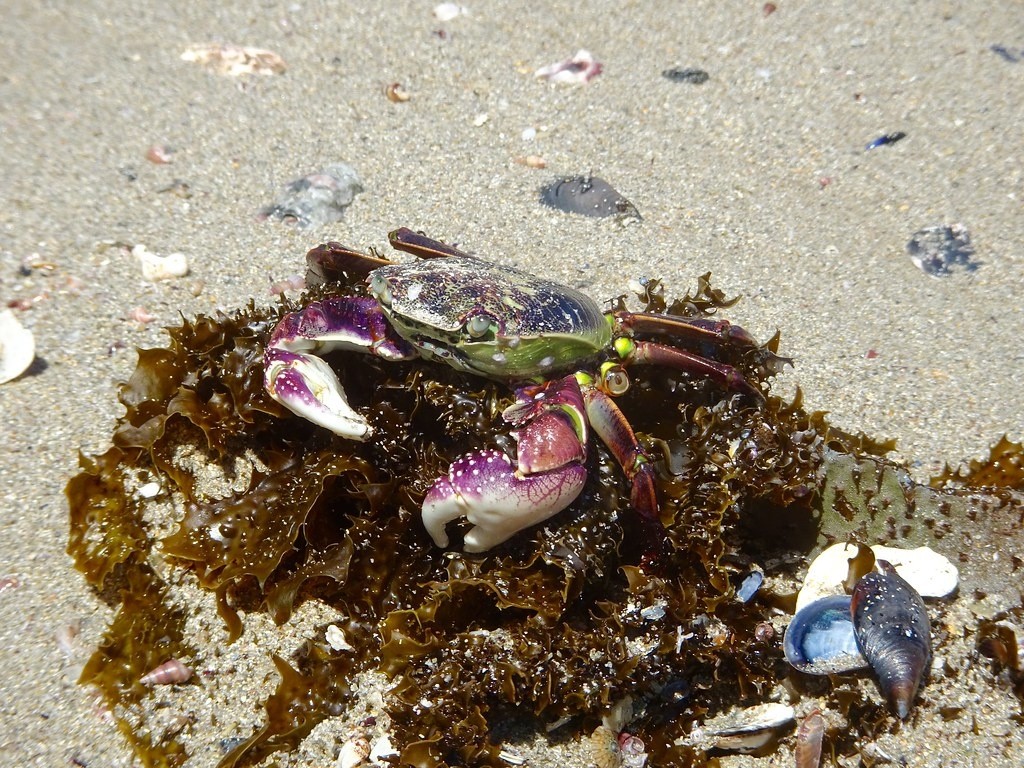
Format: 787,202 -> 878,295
268,225 -> 794,554
